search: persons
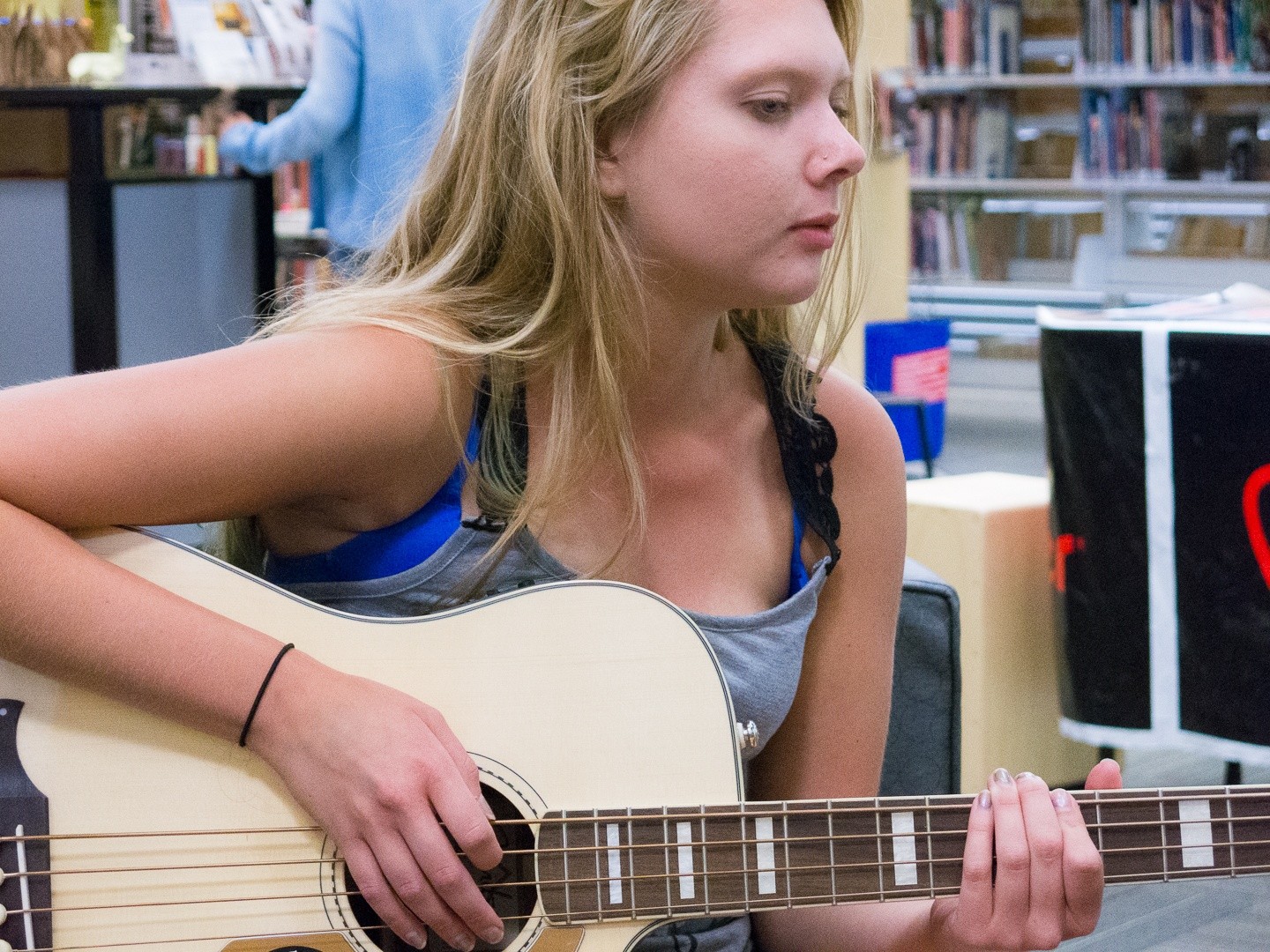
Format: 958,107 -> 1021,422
2,2 -> 1120,952
204,1 -> 502,285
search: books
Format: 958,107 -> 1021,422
909,0 -> 1270,80
897,87 -> 1210,180
909,193 -> 1009,286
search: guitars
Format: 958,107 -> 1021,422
1,526 -> 1270,952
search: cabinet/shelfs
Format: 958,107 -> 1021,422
890,72 -> 1270,305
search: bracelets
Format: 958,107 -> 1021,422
235,643 -> 293,747
